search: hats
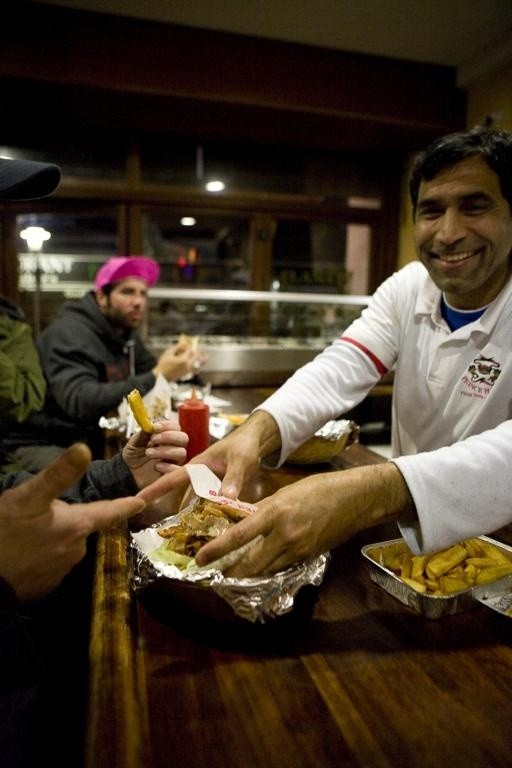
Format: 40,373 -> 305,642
0,156 -> 62,202
95,255 -> 160,294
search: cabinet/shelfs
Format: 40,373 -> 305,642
142,282 -> 396,387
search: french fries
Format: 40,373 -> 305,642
128,387 -> 155,435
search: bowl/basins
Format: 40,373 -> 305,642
232,416 -> 351,464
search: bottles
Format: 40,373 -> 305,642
177,387 -> 211,457
